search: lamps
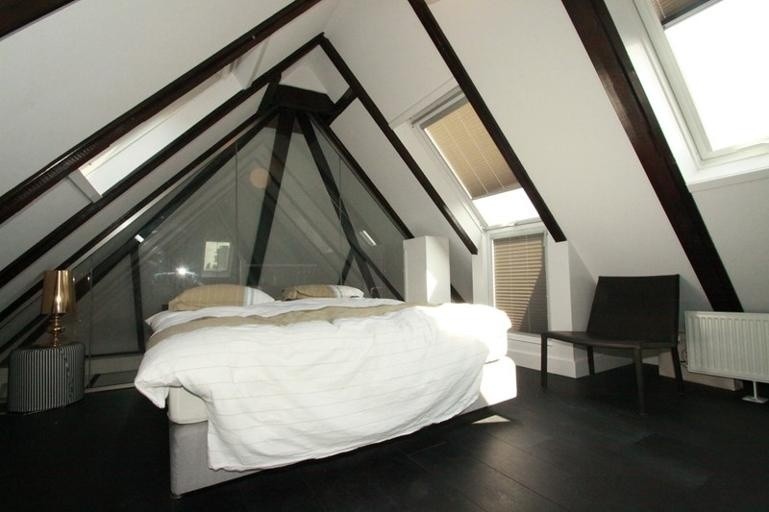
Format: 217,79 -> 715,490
41,269 -> 75,348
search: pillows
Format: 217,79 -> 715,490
169,283 -> 366,313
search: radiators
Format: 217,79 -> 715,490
686,311 -> 769,387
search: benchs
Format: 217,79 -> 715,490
536,274 -> 683,416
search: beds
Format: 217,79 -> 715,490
148,284 -> 518,500
